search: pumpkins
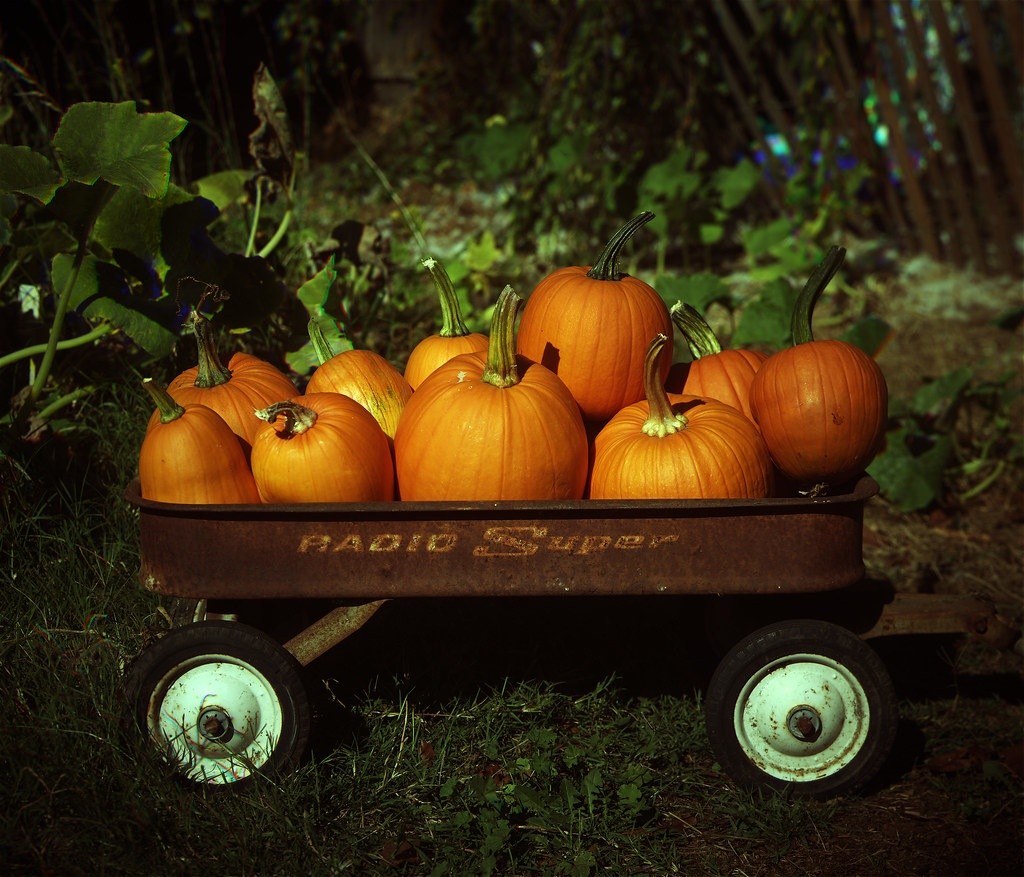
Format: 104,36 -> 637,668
137,210 -> 889,505
